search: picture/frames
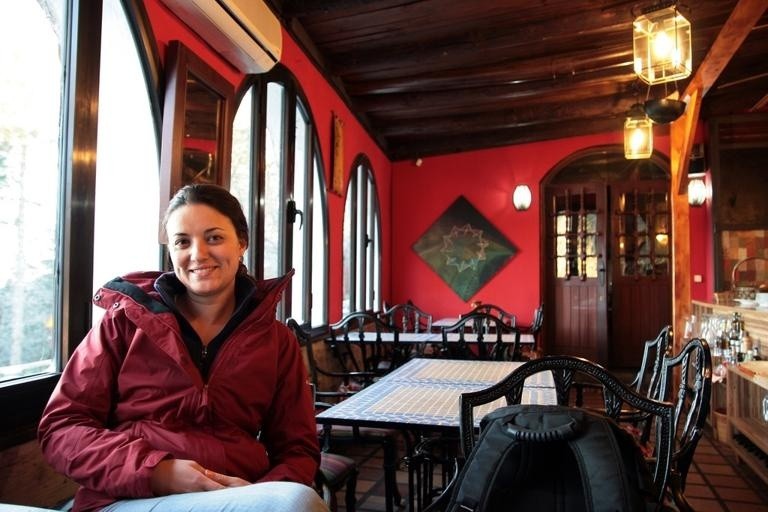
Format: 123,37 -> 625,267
157,41 -> 237,245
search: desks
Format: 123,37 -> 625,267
314,357 -> 560,508
327,329 -> 536,383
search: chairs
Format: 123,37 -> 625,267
459,303 -> 515,357
440,313 -> 522,367
430,355 -> 676,508
380,303 -> 433,357
586,326 -> 669,449
288,317 -> 414,508
521,307 -> 545,357
654,337 -> 713,505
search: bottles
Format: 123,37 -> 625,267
681,311 -> 761,366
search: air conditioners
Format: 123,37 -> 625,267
158,1 -> 283,74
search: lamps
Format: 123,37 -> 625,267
512,184 -> 533,211
632,0 -> 692,85
622,85 -> 654,159
686,178 -> 707,206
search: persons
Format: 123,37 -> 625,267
38,184 -> 330,512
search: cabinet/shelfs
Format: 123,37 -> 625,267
726,361 -> 768,487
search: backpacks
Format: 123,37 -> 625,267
449,405 -> 657,512
